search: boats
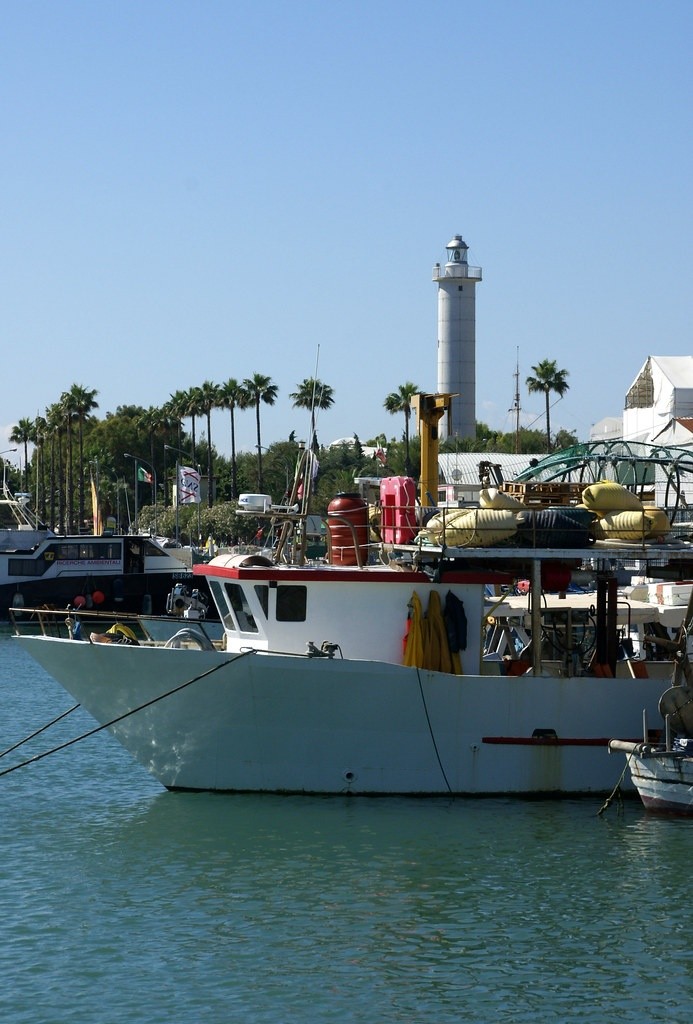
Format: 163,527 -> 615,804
0,534 -> 195,620
607,590 -> 693,811
12,506 -> 693,794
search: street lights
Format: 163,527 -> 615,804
254,444 -> 289,502
165,445 -> 201,552
124,454 -> 158,535
88,461 -> 120,534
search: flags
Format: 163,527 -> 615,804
138,467 -> 154,484
178,466 -> 202,504
296,450 -> 320,501
375,439 -> 388,467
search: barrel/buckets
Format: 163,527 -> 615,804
328,494 -> 368,566
